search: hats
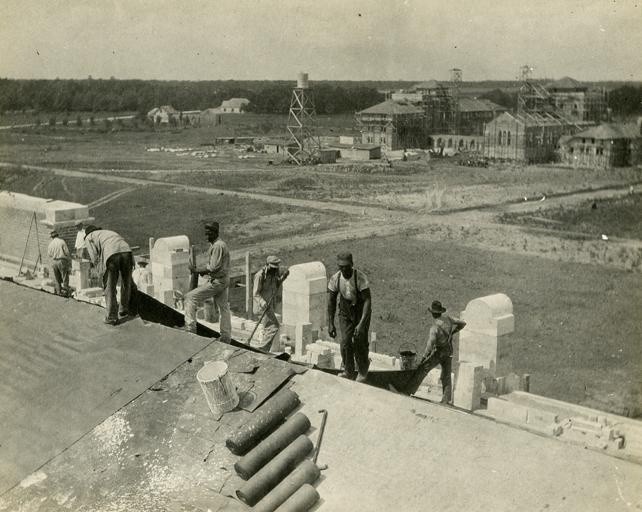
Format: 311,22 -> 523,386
203,219 -> 220,233
83,224 -> 103,240
335,250 -> 353,267
49,230 -> 59,238
266,255 -> 281,264
427,300 -> 447,314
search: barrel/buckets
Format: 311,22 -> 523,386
197,362 -> 239,415
398,343 -> 418,370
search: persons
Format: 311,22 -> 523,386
172,221 -> 233,345
74,220 -> 88,259
392,298 -> 467,405
47,229 -> 72,296
83,224 -> 136,325
326,248 -> 375,382
252,255 -> 289,353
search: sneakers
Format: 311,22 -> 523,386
174,324 -> 198,335
211,335 -> 232,345
119,310 -> 131,318
337,369 -> 357,380
355,370 -> 368,382
101,316 -> 119,326
